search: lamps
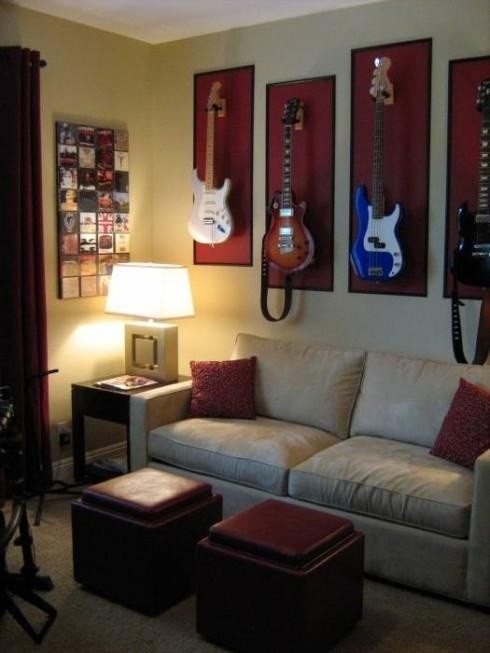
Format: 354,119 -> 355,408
102,260 -> 197,388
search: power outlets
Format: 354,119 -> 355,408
55,419 -> 72,448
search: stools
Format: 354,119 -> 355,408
192,493 -> 367,650
69,464 -> 226,619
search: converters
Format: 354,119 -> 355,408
60,432 -> 70,446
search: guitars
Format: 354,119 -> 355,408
187,82 -> 233,245
450,79 -> 490,288
264,96 -> 315,273
350,55 -> 405,283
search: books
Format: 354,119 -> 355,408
92,375 -> 159,390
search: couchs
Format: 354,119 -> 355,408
123,330 -> 488,615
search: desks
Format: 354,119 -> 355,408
68,370 -> 192,485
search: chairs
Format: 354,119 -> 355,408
1,390 -> 57,650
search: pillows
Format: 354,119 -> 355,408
426,371 -> 489,471
185,350 -> 259,422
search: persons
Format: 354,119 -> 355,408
59,189 -> 78,212
99,192 -> 112,209
60,122 -> 75,145
59,167 -> 77,190
79,171 -> 95,190
